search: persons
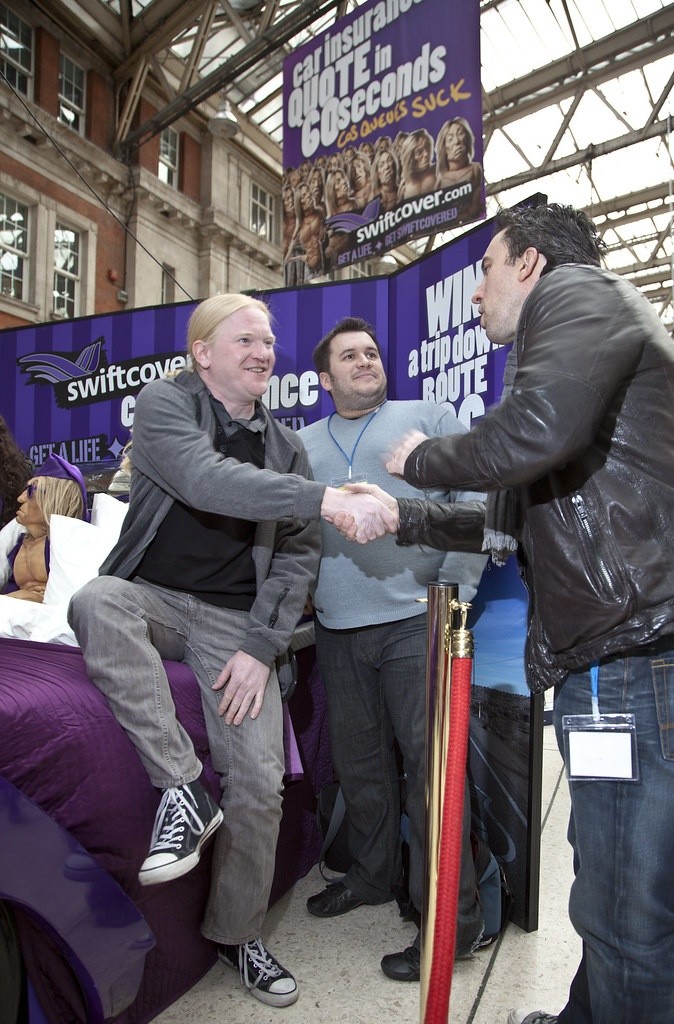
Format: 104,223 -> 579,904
296,318 -> 490,982
282,116 -> 482,272
324,203 -> 674,1024
0,416 -> 90,602
68,293 -> 398,1006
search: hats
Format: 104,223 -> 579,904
33,453 -> 91,523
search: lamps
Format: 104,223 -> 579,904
208,100 -> 242,138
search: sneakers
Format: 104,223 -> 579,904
381,944 -> 421,981
138,778 -> 224,886
507,1006 -> 559,1024
305,881 -> 394,917
218,936 -> 299,1006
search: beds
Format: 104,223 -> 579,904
0,643 -> 336,1024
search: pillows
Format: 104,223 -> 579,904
0,493 -> 129,647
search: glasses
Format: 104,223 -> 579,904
20,480 -> 47,498
